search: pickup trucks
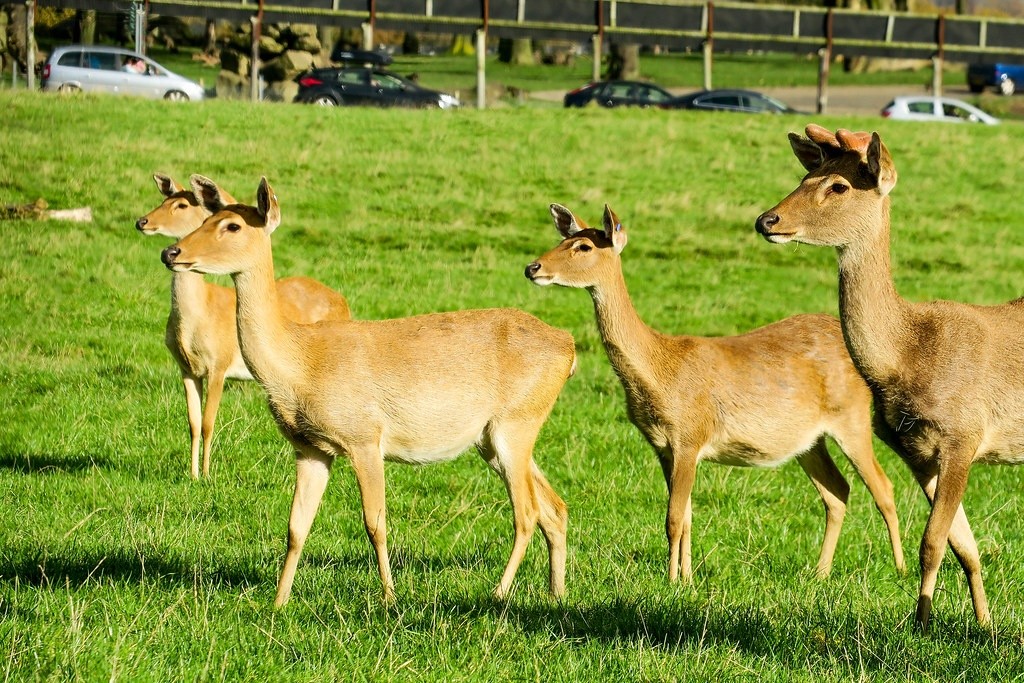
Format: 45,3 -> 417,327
329,39 -> 393,69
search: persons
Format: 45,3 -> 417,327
122,56 -> 156,75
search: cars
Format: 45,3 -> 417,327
674,90 -> 809,115
881,97 -> 1000,124
292,68 -> 460,111
565,81 -> 682,111
39,46 -> 207,105
966,52 -> 1024,95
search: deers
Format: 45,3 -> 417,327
162,172 -> 577,614
523,203 -> 906,586
755,123 -> 1023,637
135,174 -> 351,480
